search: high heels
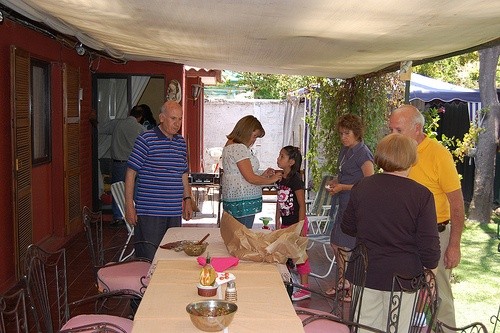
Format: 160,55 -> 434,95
340,293 -> 352,303
326,283 -> 350,297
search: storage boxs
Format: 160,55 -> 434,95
189,173 -> 218,183
213,177 -> 220,184
188,176 -> 193,183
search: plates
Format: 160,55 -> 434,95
216,273 -> 235,286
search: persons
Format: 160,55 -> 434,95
388,105 -> 465,333
124,100 -> 193,262
222,115 -> 283,229
277,146 -> 311,301
341,132 -> 441,333
323,114 -> 375,304
101,104 -> 156,225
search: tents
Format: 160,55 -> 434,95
282,71 -> 482,213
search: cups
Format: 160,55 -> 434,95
197,284 -> 218,297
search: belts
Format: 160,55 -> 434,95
113,159 -> 127,163
438,219 -> 449,225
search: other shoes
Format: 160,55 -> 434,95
110,219 -> 123,227
411,313 -> 426,327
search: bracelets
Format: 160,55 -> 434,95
183,197 -> 192,201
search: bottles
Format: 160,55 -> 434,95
200,258 -> 216,286
224,281 -> 237,303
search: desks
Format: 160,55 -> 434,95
189,182 -> 219,217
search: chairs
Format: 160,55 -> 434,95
284,240 -> 369,333
306,233 -> 337,279
302,267 -> 442,333
110,181 -> 137,263
305,175 -> 339,250
0,288 -> 28,333
81,205 -> 159,320
22,243 -> 144,333
435,319 -> 488,333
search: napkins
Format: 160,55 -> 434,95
215,271 -> 237,286
197,255 -> 240,273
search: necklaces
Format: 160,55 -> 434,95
338,152 -> 357,172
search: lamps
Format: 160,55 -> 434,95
74,44 -> 86,56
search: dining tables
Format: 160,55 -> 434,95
147,226 -> 292,283
129,261 -> 306,333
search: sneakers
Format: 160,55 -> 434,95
291,283 -> 311,301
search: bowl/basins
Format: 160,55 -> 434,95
186,299 -> 238,331
181,240 -> 209,256
